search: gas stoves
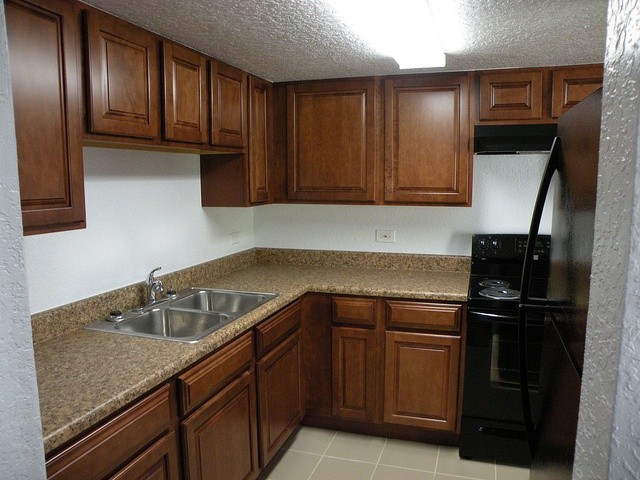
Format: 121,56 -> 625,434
467,233 -> 560,310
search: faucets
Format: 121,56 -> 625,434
146,267 -> 164,300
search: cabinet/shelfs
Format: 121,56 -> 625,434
81,3 -> 160,152
473,63 -> 603,131
382,329 -> 462,432
45,371 -> 181,479
160,37 -> 247,153
279,70 -> 473,208
3,1 -> 87,237
175,294 -> 307,480
331,296 -> 377,327
200,71 -> 279,208
385,297 -> 463,333
330,324 -> 380,425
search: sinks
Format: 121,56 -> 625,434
168,290 -> 266,313
113,307 -> 230,339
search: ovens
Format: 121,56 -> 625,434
459,311 -> 572,467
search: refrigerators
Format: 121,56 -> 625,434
516,86 -> 603,480
